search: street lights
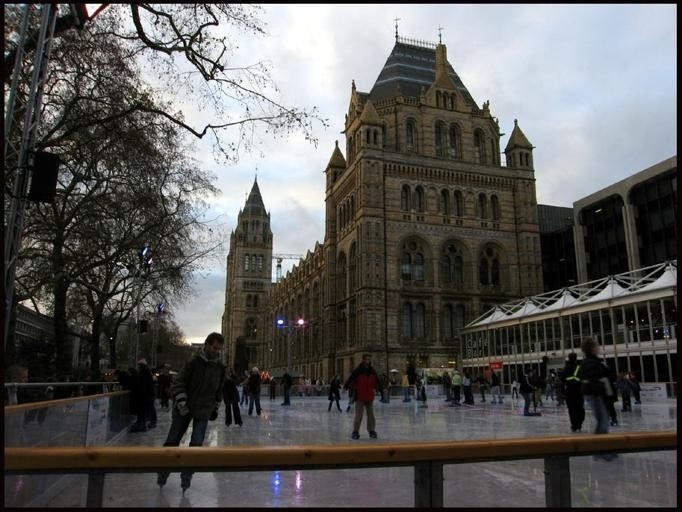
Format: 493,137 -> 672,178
155,303 -> 164,368
276,319 -> 306,378
2,0 -> 92,353
135,247 -> 153,370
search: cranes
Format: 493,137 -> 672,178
271,253 -> 305,284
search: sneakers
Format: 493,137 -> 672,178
209,401 -> 222,422
157,475 -> 167,487
369,431 -> 377,438
352,432 -> 360,440
180,478 -> 191,490
173,392 -> 189,416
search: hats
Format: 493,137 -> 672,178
137,359 -> 147,365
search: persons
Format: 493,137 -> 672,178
580,338 -> 618,461
618,372 -> 640,413
223,365 -> 343,426
342,354 -> 387,440
465,333 -> 614,358
380,372 -> 565,416
157,333 -> 226,489
12,359 -> 171,432
560,352 -> 585,433
